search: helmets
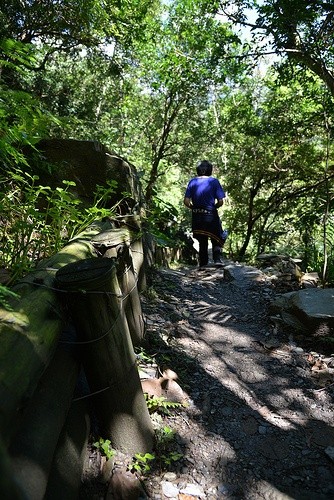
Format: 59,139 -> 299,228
196,160 -> 212,176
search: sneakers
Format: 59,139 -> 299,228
214,260 -> 225,265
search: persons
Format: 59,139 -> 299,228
184,160 -> 226,266
183,237 -> 198,265
